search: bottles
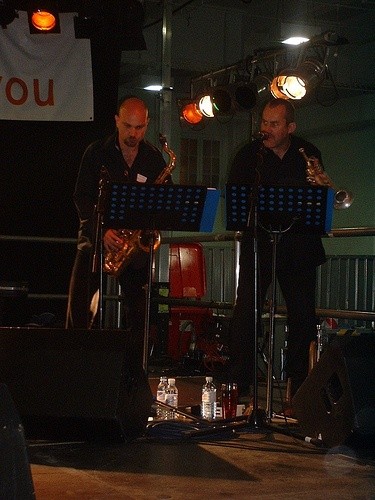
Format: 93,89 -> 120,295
201,376 -> 218,420
164,378 -> 178,420
156,376 -> 169,420
219,383 -> 238,419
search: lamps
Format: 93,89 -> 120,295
0,0 -> 62,35
176,44 -> 329,129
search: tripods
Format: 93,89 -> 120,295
181,138 -> 335,447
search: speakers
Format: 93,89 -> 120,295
0,327 -> 153,499
288,335 -> 375,450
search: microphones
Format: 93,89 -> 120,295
257,131 -> 270,139
100,162 -> 107,174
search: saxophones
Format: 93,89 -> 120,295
102,133 -> 178,276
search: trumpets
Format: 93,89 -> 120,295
298,147 -> 353,210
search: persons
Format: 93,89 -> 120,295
218,98 -> 328,401
65,95 -> 178,368
